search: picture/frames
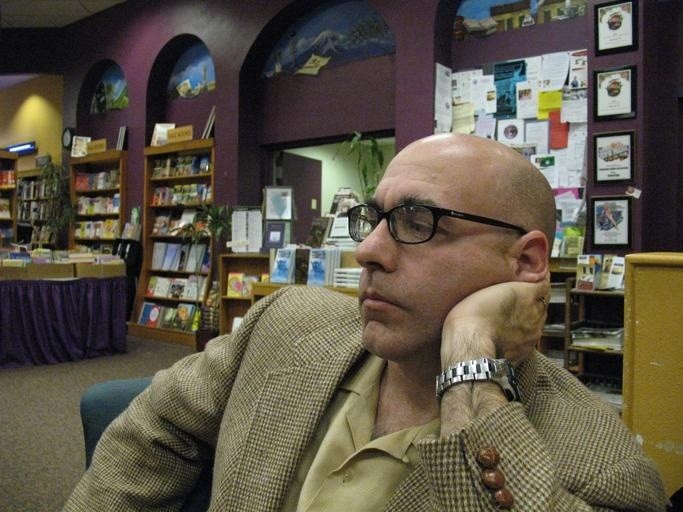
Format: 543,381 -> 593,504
591,129 -> 637,185
590,195 -> 633,250
593,64 -> 637,122
594,0 -> 638,57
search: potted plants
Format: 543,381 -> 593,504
169,200 -> 232,352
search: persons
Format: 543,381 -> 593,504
61,133 -> 671,510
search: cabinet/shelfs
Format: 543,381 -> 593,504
219,252 -> 363,335
564,276 -> 625,417
536,268 -> 585,377
112,238 -> 141,322
15,165 -> 61,251
127,137 -> 216,347
69,150 -> 127,254
0,150 -> 17,250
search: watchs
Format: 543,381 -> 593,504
433,354 -> 520,404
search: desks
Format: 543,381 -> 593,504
0,275 -> 128,369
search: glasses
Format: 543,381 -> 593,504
344,200 -> 527,245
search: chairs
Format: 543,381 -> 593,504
80,378 -> 214,512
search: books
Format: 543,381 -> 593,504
540,252 -> 624,410
226,185 -> 297,330
71,127 -> 141,259
297,192 -> 363,288
0,167 -> 52,247
1,244 -> 125,279
135,104 -> 216,330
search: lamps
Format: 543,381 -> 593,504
8,143 -> 32,152
